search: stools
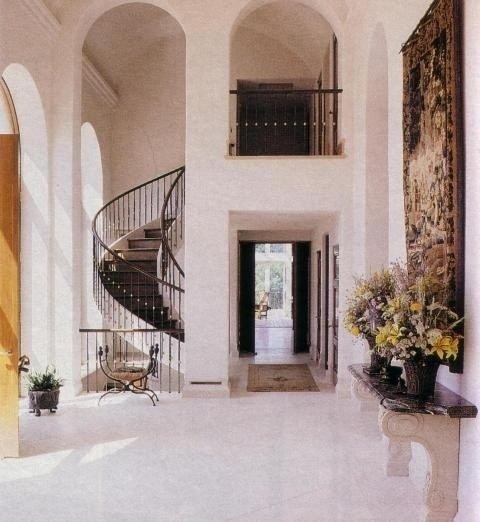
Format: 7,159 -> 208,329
95,342 -> 161,406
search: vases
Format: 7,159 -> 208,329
362,335 -> 439,398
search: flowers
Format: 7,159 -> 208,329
341,256 -> 460,360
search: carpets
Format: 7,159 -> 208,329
245,363 -> 321,393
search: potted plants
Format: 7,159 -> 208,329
23,364 -> 64,419
